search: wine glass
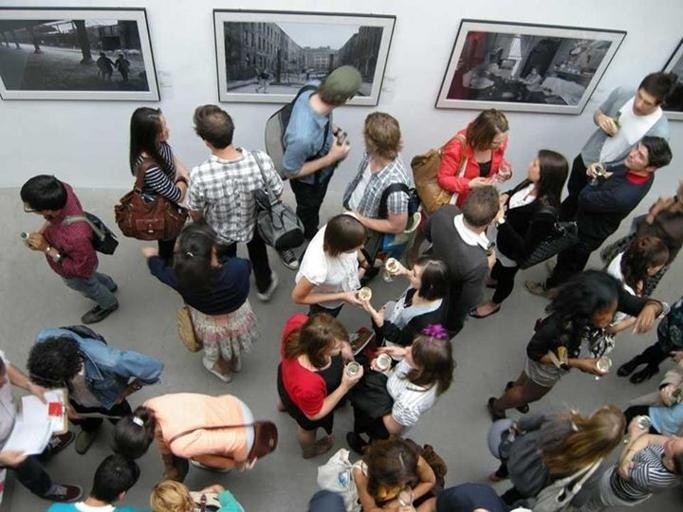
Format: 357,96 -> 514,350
381,257 -> 398,283
593,354 -> 613,382
588,162 -> 606,185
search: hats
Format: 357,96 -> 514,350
325,64 -> 362,96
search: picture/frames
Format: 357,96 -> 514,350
212,9 -> 396,107
435,18 -> 627,115
659,39 -> 683,121
0,6 -> 162,102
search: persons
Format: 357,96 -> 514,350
485,61 -> 509,95
128,107 -> 192,260
254,68 -> 272,95
93,49 -> 115,80
14,173 -> 121,322
112,53 -> 132,82
278,64 -> 364,236
0,70 -> 683,512
516,63 -> 542,101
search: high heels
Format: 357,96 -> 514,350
617,355 -> 641,377
489,397 -> 506,423
506,381 -> 529,413
630,363 -> 659,383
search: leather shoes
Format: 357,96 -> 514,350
469,300 -> 500,319
486,278 -> 496,287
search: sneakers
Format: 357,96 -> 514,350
525,280 -> 547,296
303,436 -> 335,459
202,358 -> 231,384
74,430 -> 94,454
347,433 -> 368,455
48,429 -> 74,453
491,473 -> 501,480
81,301 -> 119,324
546,260 -> 555,272
277,250 -> 299,269
255,271 -> 279,302
41,485 -> 83,502
232,354 -> 242,372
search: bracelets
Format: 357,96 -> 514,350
42,244 -> 57,259
174,176 -> 188,187
332,123 -> 343,137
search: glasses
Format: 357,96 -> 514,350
22,203 -> 38,212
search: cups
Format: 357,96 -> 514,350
395,485 -> 416,509
376,353 -> 391,372
356,287 -> 373,303
495,159 -> 513,175
636,415 -> 652,430
345,360 -> 360,377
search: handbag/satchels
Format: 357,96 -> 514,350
55,323 -> 106,345
257,200 -> 306,249
249,422 -> 277,463
115,189 -> 185,241
350,327 -> 385,364
86,212 -> 118,254
316,449 -> 366,512
176,306 -> 203,352
534,477 -> 574,511
411,149 -> 454,215
487,420 -> 512,458
520,222 -> 577,269
382,212 -> 421,252
265,104 -> 290,180
